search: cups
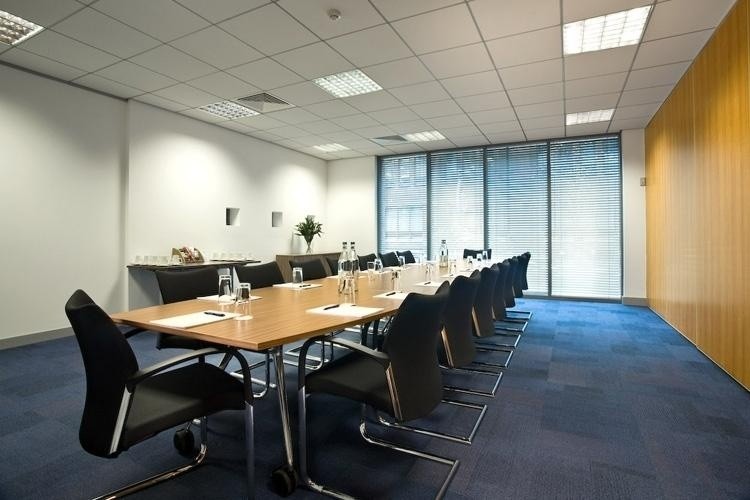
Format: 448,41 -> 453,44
235,282 -> 253,321
451,249 -> 488,274
218,275 -> 233,305
339,275 -> 355,309
291,267 -> 304,289
367,254 -> 433,293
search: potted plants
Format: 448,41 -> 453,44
294,216 -> 323,255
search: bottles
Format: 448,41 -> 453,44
439,240 -> 449,268
337,240 -> 359,293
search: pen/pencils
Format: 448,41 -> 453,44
299,284 -> 311,287
323,304 -> 340,310
386,291 -> 396,296
204,311 -> 225,316
424,281 -> 431,284
450,274 -> 453,276
